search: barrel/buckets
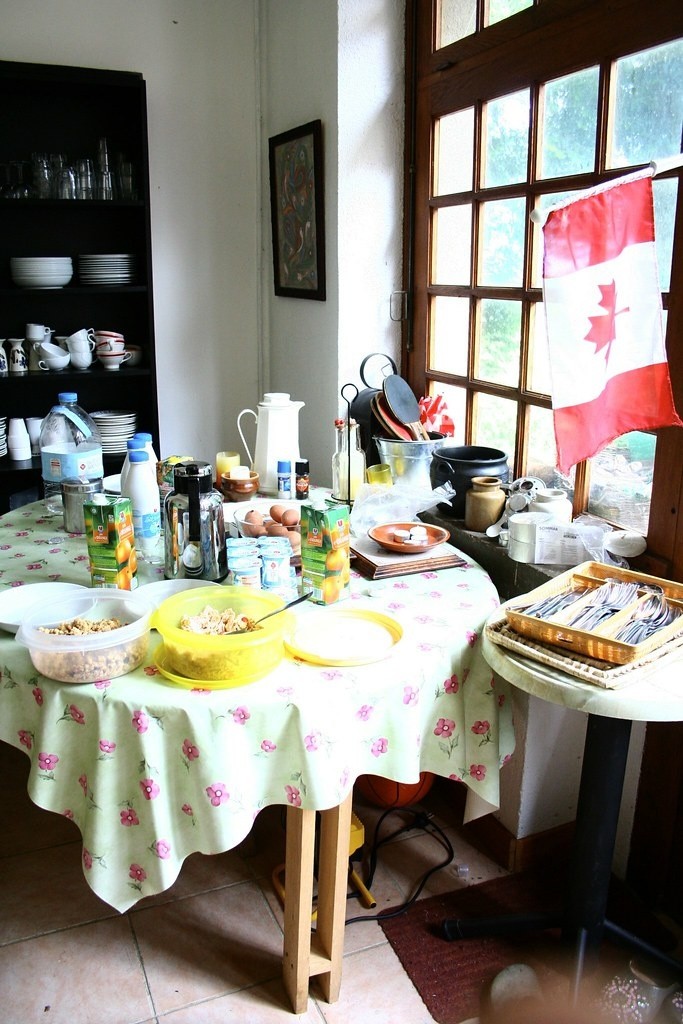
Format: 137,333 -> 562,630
373,431 -> 448,485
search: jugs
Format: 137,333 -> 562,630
237,393 -> 305,496
164,461 -> 229,583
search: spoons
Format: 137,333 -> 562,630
222,591 -> 314,635
610,596 -> 683,644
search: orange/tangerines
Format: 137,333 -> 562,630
114,540 -> 137,590
320,548 -> 350,605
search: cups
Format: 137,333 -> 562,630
0,136 -> 140,200
27,324 -> 50,341
216,452 -> 240,484
8,418 -> 31,460
367,464 -> 393,492
33,328 -> 95,371
30,333 -> 51,370
88,331 -> 142,369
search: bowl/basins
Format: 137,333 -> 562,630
15,588 -> 294,683
234,506 -> 302,556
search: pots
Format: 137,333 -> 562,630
430,446 -> 509,520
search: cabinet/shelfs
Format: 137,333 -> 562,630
0,59 -> 160,515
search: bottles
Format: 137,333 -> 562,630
278,460 -> 310,500
0,339 -> 28,372
39,393 -> 161,550
466,446 -> 573,533
61,476 -> 104,534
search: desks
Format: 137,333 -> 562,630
0,471 -> 513,1013
436,595 -> 683,1023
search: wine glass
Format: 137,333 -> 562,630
54,337 -> 71,368
26,418 -> 44,456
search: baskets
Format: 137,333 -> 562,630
505,559 -> 683,665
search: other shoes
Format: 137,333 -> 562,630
594,958 -> 681,1024
488,963 -> 552,1024
668,991 -> 683,1024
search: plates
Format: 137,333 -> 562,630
103,474 -> 122,494
0,579 -> 223,634
10,257 -> 73,289
78,255 -> 132,284
368,522 -> 450,553
0,417 -> 7,457
89,410 -> 136,453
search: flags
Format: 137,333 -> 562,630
540,166 -> 682,477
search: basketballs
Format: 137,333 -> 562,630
355,771 -> 435,808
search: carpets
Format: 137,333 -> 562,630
378,864 -> 603,1024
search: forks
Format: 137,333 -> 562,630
566,582 -> 639,632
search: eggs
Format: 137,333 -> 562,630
242,504 -> 303,549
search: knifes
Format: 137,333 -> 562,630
522,586 -> 597,619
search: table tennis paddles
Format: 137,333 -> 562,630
369,374 -> 432,441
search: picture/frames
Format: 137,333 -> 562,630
267,118 -> 326,299
